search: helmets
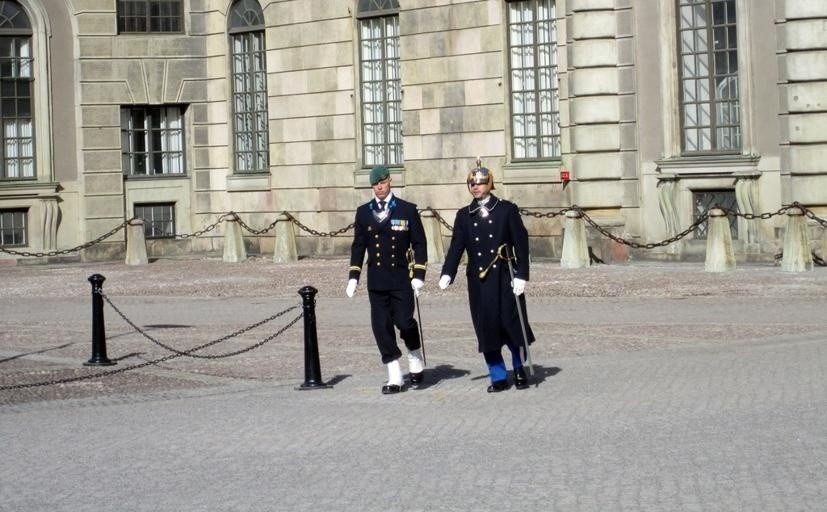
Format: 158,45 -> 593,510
466,155 -> 496,191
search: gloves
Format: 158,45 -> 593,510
509,278 -> 526,297
438,274 -> 451,290
345,278 -> 358,298
410,278 -> 425,291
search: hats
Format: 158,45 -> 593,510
368,166 -> 389,187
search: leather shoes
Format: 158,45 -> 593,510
486,380 -> 509,393
408,368 -> 425,389
380,381 -> 405,394
512,366 -> 528,390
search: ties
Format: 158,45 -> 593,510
378,201 -> 386,212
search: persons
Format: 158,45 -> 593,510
439,166 -> 534,391
345,167 -> 426,395
